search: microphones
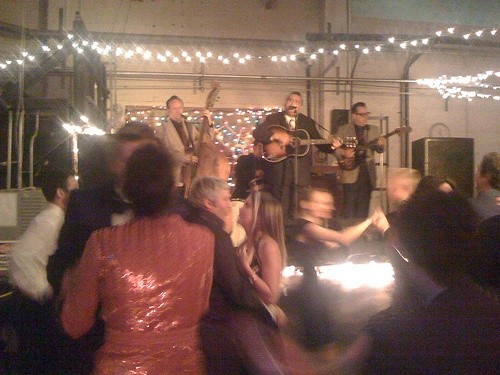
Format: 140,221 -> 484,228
288,106 -> 298,110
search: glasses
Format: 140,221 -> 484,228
355,112 -> 371,117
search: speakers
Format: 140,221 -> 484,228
411,137 -> 474,198
330,110 -> 350,134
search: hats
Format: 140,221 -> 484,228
253,293 -> 289,329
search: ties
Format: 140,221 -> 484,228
289,119 -> 294,131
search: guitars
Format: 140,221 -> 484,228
336,125 -> 414,170
261,124 -> 357,165
181,80 -> 218,192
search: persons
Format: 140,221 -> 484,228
253,90 -> 343,220
285,182 -> 375,361
59,143 -> 216,374
331,100 -> 386,218
319,148 -> 499,374
374,167 -> 426,307
163,95 -> 216,185
11,119 -> 289,374
233,140 -> 273,201
